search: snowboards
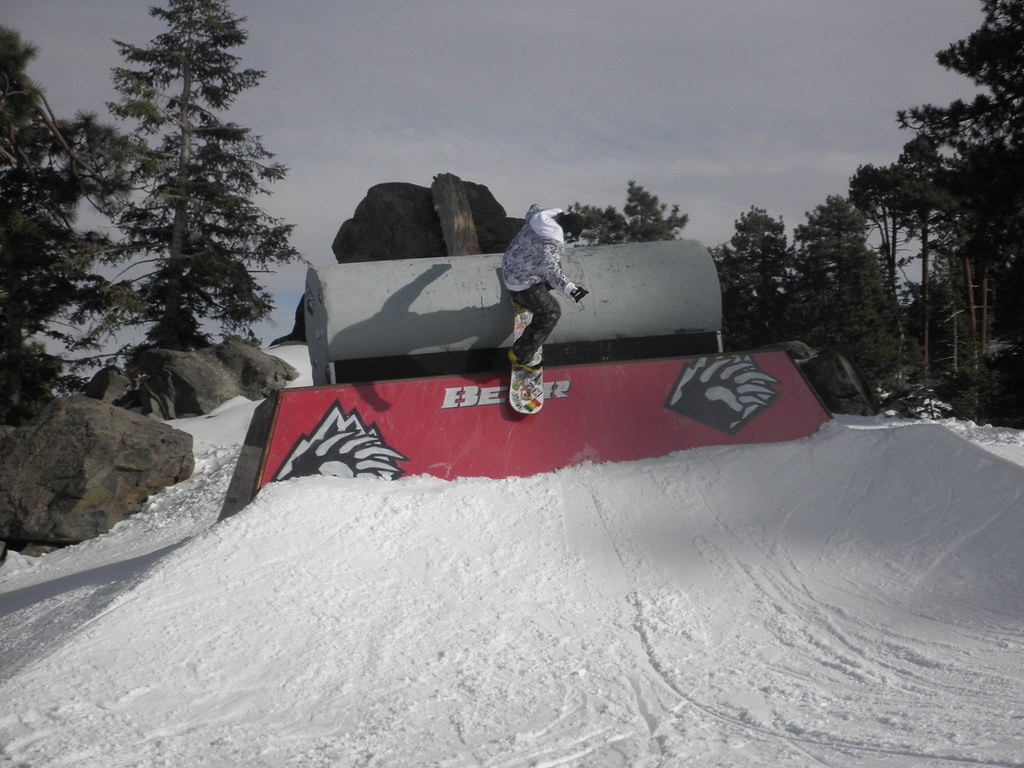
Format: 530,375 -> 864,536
509,302 -> 545,415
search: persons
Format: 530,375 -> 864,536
501,204 -> 590,371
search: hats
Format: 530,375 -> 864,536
560,214 -> 583,242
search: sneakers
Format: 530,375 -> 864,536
509,347 -> 544,369
513,298 -> 529,313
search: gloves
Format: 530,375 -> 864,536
569,286 -> 589,302
